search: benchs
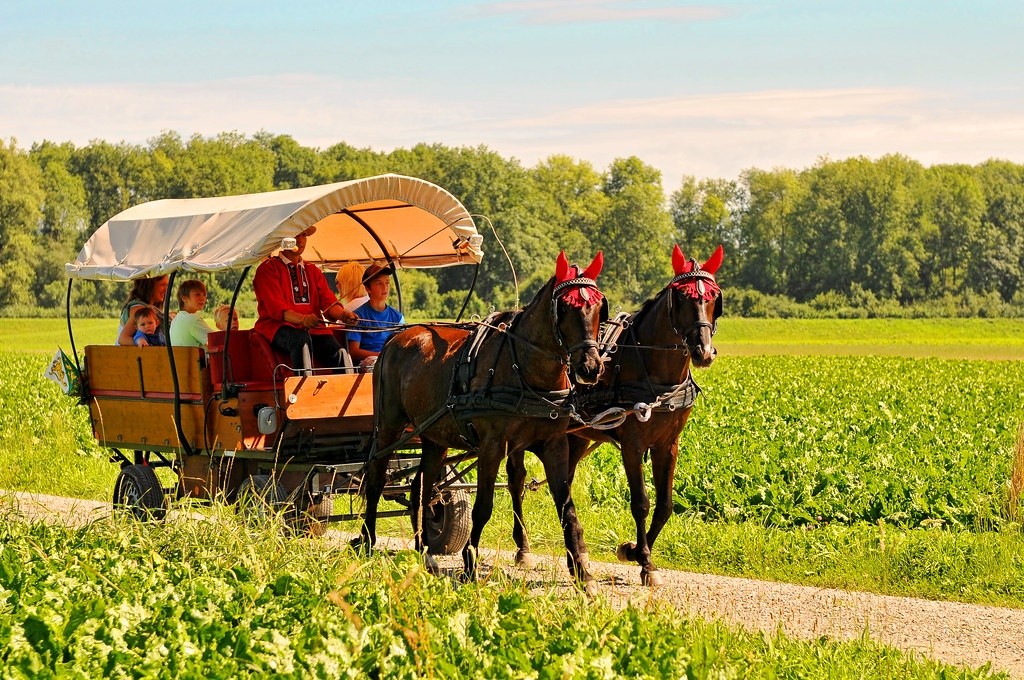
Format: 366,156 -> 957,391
205,325 -> 348,451
85,345 -> 212,449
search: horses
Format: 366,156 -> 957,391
349,250 -> 604,599
507,242 -> 724,588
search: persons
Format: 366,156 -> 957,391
330,262 -> 406,369
115,274 -> 176,350
253,226 -> 358,375
213,305 -> 240,331
169,280 -> 213,347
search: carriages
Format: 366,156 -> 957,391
47,174 -> 725,585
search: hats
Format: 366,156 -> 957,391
306,226 -> 317,236
362,265 -> 393,286
